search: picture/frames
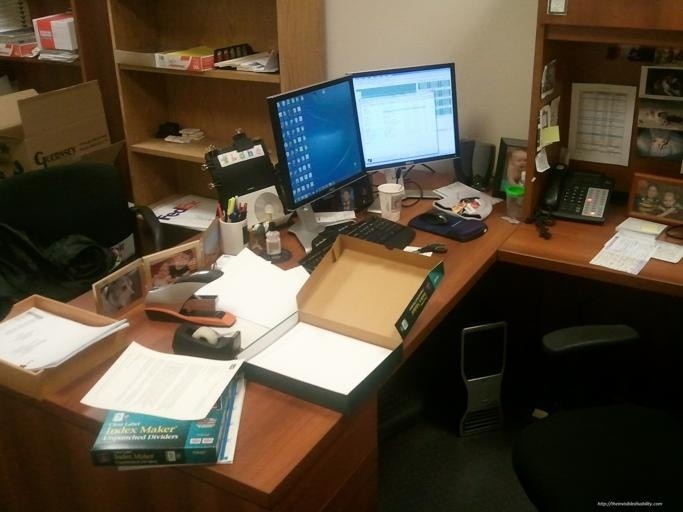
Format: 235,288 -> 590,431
638,65 -> 683,102
568,82 -> 637,168
142,240 -> 201,298
625,171 -> 683,226
92,258 -> 146,319
492,136 -> 528,199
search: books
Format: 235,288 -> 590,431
614,216 -> 668,239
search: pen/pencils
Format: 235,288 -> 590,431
217,196 -> 247,223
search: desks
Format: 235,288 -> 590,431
1,168 -> 534,511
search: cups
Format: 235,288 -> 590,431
377,183 -> 405,223
217,215 -> 249,255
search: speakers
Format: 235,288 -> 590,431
454,139 -> 496,190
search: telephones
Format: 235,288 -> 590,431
539,163 -> 615,224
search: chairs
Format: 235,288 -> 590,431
0,163 -> 164,324
506,322 -> 683,511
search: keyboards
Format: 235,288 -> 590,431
301,212 -> 415,290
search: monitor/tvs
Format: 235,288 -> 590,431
350,62 -> 462,196
267,76 -> 366,255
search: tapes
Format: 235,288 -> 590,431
192,326 -> 236,344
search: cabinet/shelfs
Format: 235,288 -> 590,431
106,0 -> 327,260
0,0 -> 124,145
497,0 -> 683,301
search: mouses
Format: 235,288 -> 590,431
425,206 -> 449,225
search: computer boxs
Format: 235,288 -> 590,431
448,307 -> 513,438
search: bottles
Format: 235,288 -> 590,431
505,186 -> 526,219
264,222 -> 282,256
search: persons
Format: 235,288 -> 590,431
656,190 -> 683,222
507,149 -> 527,185
634,183 -> 663,216
167,249 -> 198,279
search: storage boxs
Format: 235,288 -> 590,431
231,233 -> 445,417
32,10 -> 79,51
0,293 -> 128,400
0,79 -> 114,178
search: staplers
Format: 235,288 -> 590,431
144,269 -> 237,328
